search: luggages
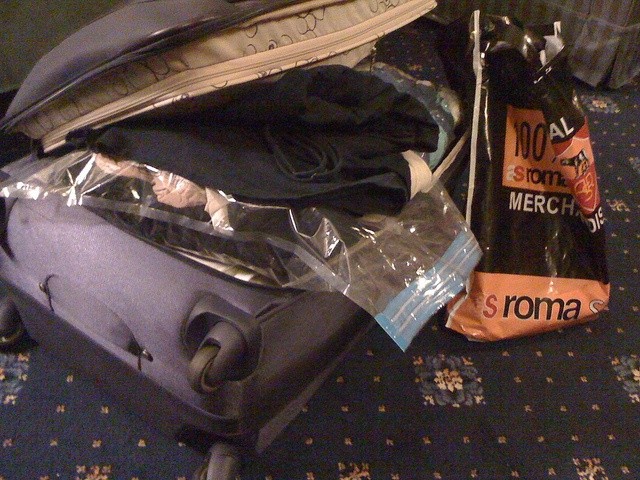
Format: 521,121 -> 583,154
0,0 -> 472,480
